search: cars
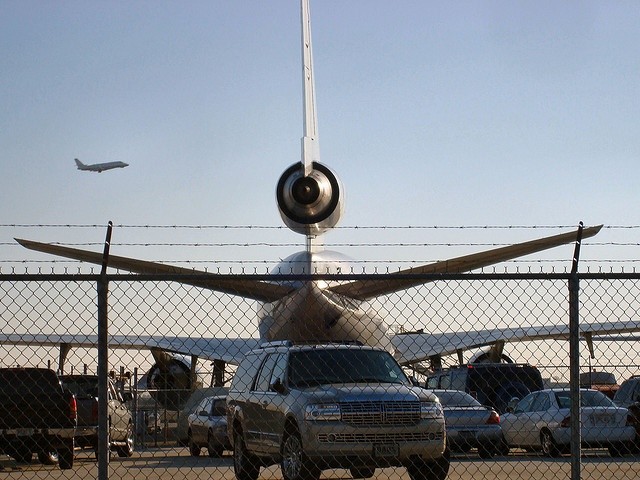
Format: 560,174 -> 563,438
425,390 -> 502,459
188,395 -> 234,458
496,388 -> 636,458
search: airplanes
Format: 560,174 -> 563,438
0,0 -> 639,366
74,159 -> 129,173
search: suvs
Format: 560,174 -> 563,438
580,372 -> 619,400
611,375 -> 640,408
226,340 -> 450,480
425,363 -> 544,417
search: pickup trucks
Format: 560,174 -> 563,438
15,375 -> 134,462
0,368 -> 77,470
